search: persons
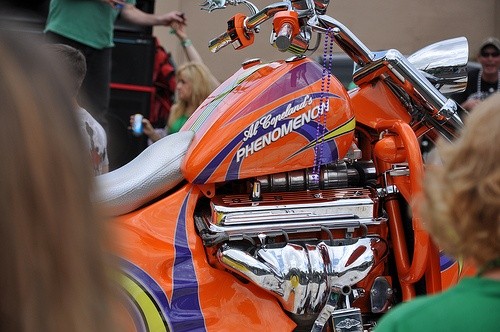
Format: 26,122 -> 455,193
0,1 -> 220,332
368,90 -> 500,332
456,37 -> 500,113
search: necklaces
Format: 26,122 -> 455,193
477,68 -> 500,99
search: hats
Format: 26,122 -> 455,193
480,37 -> 500,50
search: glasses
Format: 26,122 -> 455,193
481,51 -> 500,57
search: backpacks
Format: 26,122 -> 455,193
149,37 -> 176,129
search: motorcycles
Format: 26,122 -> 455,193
94,1 -> 483,330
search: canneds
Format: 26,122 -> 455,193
132,114 -> 144,135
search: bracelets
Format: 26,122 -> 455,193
180,39 -> 193,48
